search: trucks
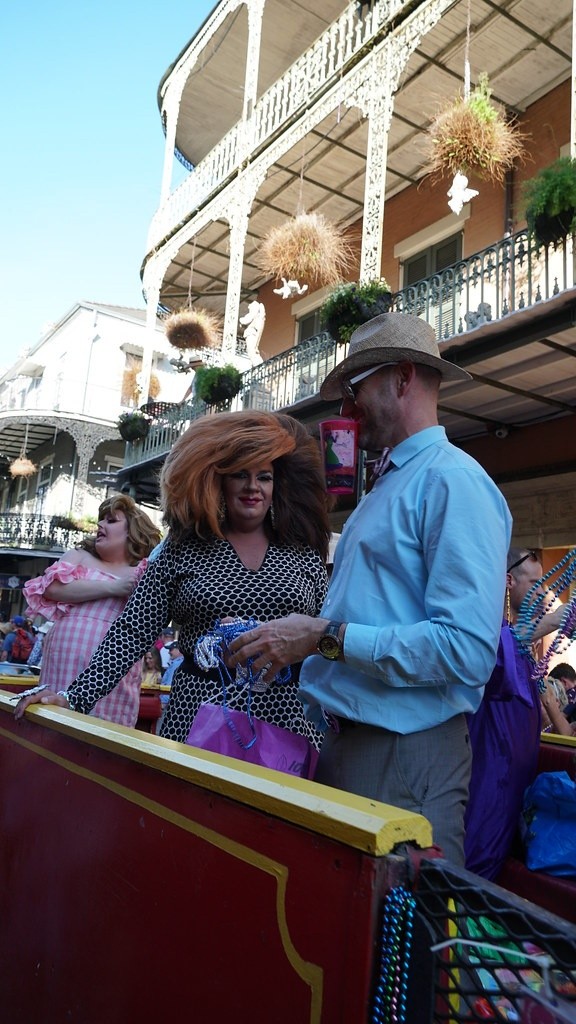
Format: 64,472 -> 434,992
0,673 -> 576,1024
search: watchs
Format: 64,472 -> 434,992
315,620 -> 345,661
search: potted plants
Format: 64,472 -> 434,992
199,359 -> 243,405
518,154 -> 576,249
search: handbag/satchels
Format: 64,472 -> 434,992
518,770 -> 576,877
184,704 -> 320,783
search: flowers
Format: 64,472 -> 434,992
115,411 -> 150,441
315,276 -> 390,343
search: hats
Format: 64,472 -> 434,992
26,617 -> 35,626
159,627 -> 175,636
9,614 -> 24,627
319,312 -> 473,402
37,621 -> 55,633
163,641 -> 179,649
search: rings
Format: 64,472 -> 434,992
9,684 -> 49,701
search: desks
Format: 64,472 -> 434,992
140,402 -> 181,418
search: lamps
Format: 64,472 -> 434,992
9,589 -> 17,603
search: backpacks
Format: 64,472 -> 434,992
465,620 -> 542,879
10,629 -> 34,660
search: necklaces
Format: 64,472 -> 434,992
369,887 -> 416,1024
509,546 -> 576,681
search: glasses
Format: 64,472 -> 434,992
340,362 -> 420,401
507,548 -> 538,574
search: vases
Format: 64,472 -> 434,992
328,292 -> 390,345
120,419 -> 149,441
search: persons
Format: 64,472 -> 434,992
156,641 -> 184,735
24,494 -> 163,728
220,313 -> 513,868
502,546 -> 568,643
140,646 -> 162,684
13,409 -> 331,744
239,301 -> 266,365
179,355 -> 207,406
0,616 -> 54,666
540,662 -> 576,738
159,627 -> 176,677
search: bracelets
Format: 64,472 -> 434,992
57,691 -> 75,710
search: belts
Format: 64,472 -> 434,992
179,653 -> 304,692
